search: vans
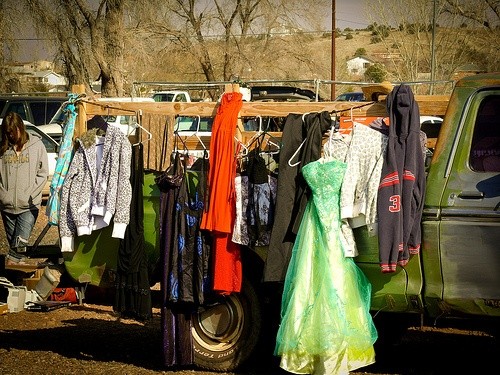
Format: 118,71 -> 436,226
49,96 -> 155,138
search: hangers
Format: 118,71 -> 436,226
76,104 -> 440,168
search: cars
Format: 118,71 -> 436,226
0,118 -> 69,203
334,92 -> 364,101
245,85 -> 323,103
417,115 -> 443,128
34,122 -> 64,146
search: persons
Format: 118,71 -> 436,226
0,112 -> 49,264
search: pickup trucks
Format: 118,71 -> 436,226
61,68 -> 499,371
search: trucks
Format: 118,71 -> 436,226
152,91 -> 208,131
